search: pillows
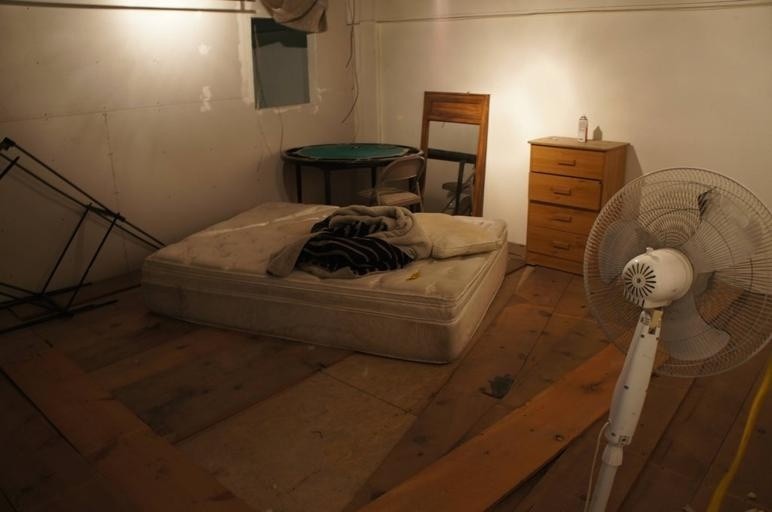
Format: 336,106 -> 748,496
414,213 -> 509,259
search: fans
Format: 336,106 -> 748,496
582,167 -> 772,512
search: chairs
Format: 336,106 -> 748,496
442,174 -> 474,212
357,154 -> 426,214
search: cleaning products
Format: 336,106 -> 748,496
576,114 -> 589,143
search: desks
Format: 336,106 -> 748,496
281,143 -> 423,205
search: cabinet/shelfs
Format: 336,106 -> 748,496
525,136 -> 629,276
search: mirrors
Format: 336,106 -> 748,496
418,90 -> 490,216
